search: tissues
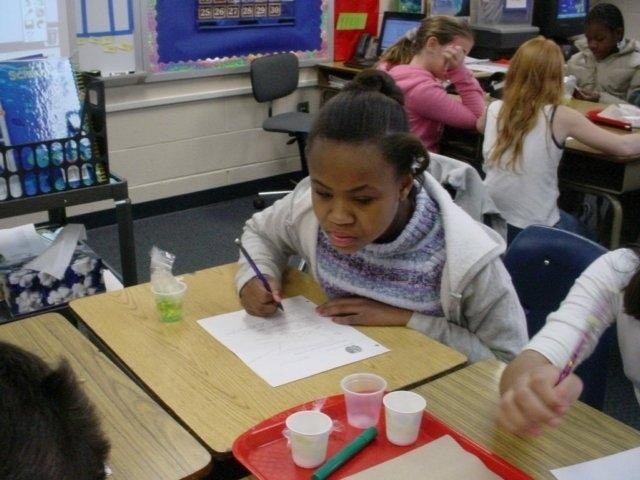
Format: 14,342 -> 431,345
0,221 -> 107,319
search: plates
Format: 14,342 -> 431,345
103,71 -> 148,88
586,108 -> 634,129
230,391 -> 532,480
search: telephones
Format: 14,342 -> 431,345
343,31 -> 380,68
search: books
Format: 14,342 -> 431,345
0,52 -> 109,203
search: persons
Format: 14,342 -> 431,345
566,0 -> 640,106
0,338 -> 111,480
235,69 -> 529,364
373,14 -> 486,151
476,34 -> 640,243
494,238 -> 640,437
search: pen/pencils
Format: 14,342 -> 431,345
234,239 -> 285,311
556,292 -> 617,386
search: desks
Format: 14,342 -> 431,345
70,260 -> 469,464
406,357 -> 624,480
557,98 -> 639,252
0,170 -> 140,288
0,311 -> 214,480
314,59 -> 499,90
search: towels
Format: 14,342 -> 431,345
349,434 -> 508,479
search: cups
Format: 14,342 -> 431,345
340,373 -> 388,429
285,410 -> 333,469
151,282 -> 187,323
382,391 -> 427,446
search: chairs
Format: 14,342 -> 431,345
250,52 -> 319,208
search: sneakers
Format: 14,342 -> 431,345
580,202 -> 598,240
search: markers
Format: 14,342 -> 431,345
311,426 -> 378,479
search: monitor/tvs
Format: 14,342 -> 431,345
375,10 -> 426,56
553,0 -> 590,22
